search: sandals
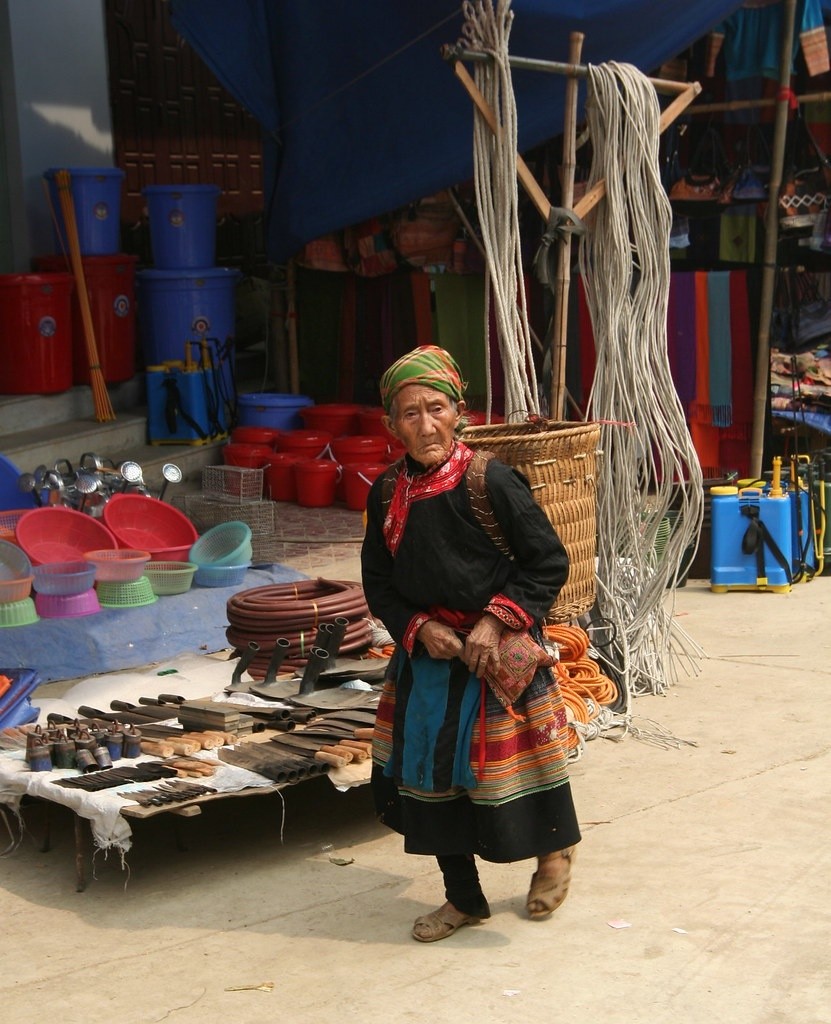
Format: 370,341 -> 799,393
412,903 -> 480,941
527,846 -> 575,918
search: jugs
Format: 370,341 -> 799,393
20,451 -> 182,528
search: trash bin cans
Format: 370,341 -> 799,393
0,163 -> 242,450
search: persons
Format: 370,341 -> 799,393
362,345 -> 582,943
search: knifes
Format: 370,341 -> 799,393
117,724 -> 237,808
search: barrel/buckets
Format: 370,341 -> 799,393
44,164 -> 123,258
224,394 -> 504,511
137,268 -> 245,403
143,184 -> 221,270
619,510 -> 695,590
39,251 -> 141,385
0,274 -> 75,394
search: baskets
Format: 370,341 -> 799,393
0,493 -> 253,629
